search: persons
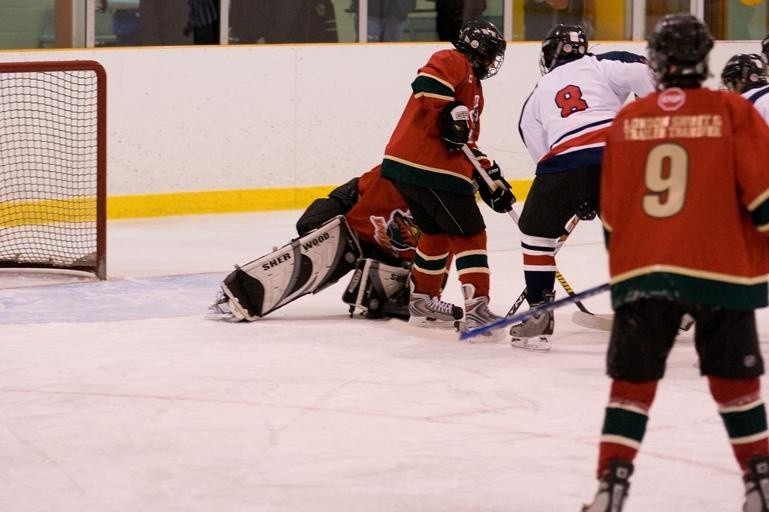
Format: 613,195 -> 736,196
581,12 -> 769,511
377,17 -> 516,335
113,1 -> 587,49
205,156 -> 479,326
718,54 -> 768,130
511,23 -> 659,340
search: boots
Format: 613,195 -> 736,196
509,290 -> 556,338
462,283 -> 506,327
408,272 -> 463,321
741,452 -> 768,511
584,459 -> 633,511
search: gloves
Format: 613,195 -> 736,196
438,102 -> 470,155
576,199 -> 596,221
474,160 -> 517,214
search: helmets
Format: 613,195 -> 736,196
452,19 -> 506,79
539,24 -> 589,74
645,11 -> 714,91
721,51 -> 769,94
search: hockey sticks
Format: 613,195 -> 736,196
572,312 -> 614,331
